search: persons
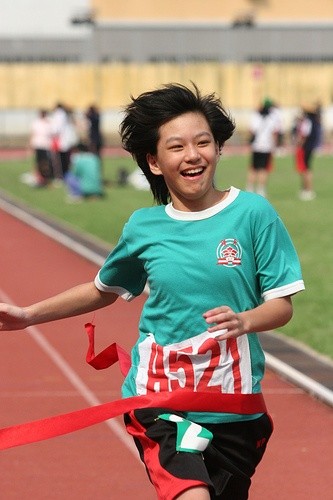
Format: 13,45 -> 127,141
237,104 -> 276,199
35,98 -> 103,182
62,143 -> 102,199
295,100 -> 317,199
260,98 -> 322,173
0,78 -> 306,500
